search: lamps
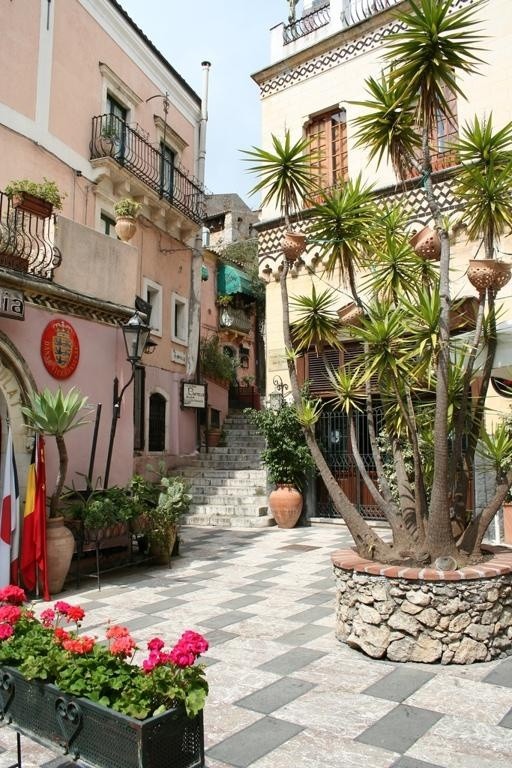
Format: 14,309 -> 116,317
160,246 -> 209,282
266,376 -> 288,414
143,333 -> 157,354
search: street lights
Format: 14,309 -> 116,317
103,309 -> 152,490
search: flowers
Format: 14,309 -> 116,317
0,585 -> 209,720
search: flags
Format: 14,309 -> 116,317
0,427 -> 21,587
20,431 -> 52,602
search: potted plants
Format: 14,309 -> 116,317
496,411 -> 512,545
90,126 -> 120,159
242,374 -> 257,393
113,199 -> 144,242
21,384 -> 193,594
4,175 -> 65,220
205,428 -> 223,447
243,380 -> 315,528
0,242 -> 29,274
